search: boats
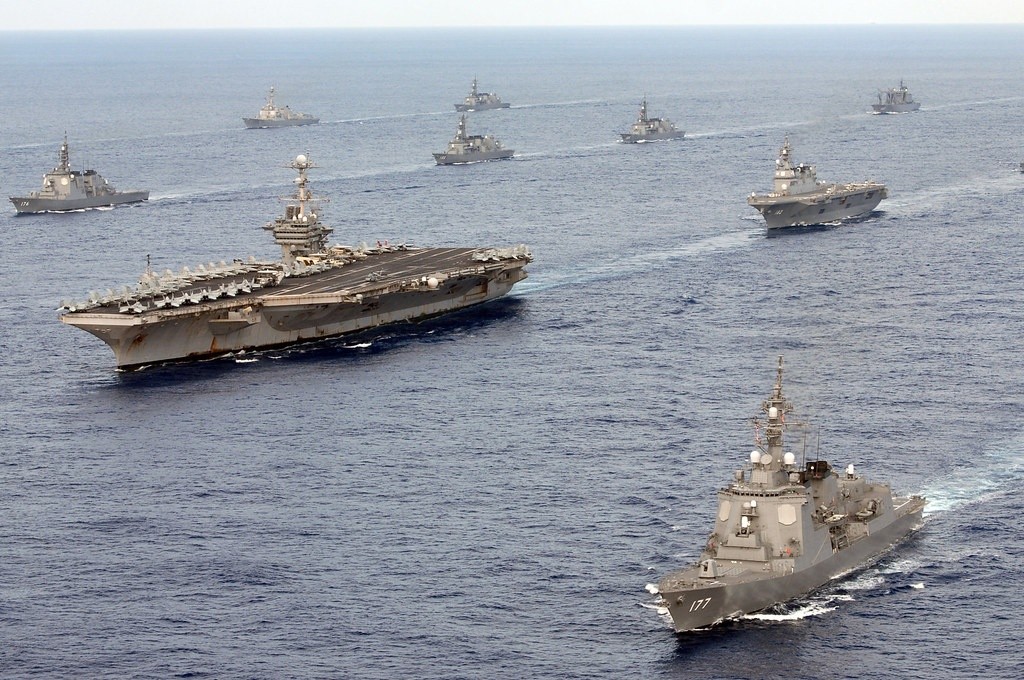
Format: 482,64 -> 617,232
454,75 -> 511,112
431,113 -> 515,165
61,147 -> 534,373
657,353 -> 927,634
242,87 -> 320,129
620,92 -> 686,143
746,131 -> 887,228
871,78 -> 922,113
8,131 -> 152,214
